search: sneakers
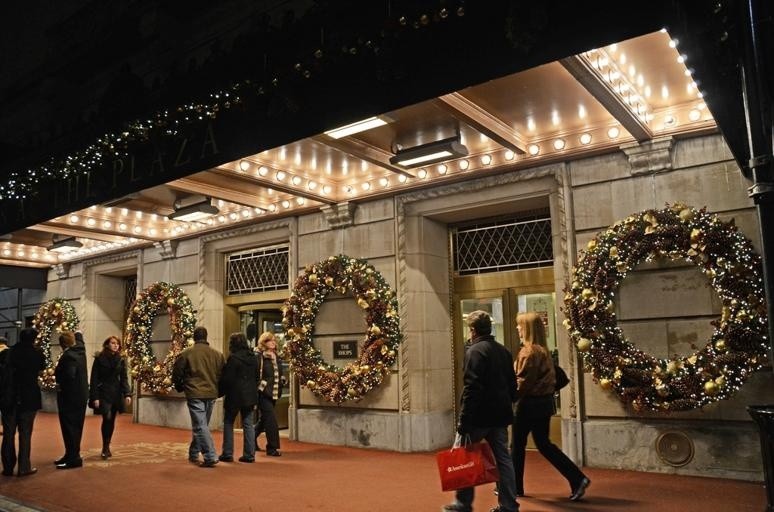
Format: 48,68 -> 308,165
53,454 -> 66,464
1,468 -> 15,477
265,447 -> 281,457
566,475 -> 591,501
238,455 -> 256,463
101,443 -> 112,460
440,501 -> 474,512
198,458 -> 220,467
17,467 -> 38,478
218,453 -> 234,462
54,457 -> 83,469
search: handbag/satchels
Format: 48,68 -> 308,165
433,431 -> 501,492
554,363 -> 570,392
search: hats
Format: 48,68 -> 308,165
460,310 -> 494,331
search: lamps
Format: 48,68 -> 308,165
387,115 -> 470,170
44,227 -> 83,254
164,189 -> 221,225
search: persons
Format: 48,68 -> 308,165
491,310 -> 590,500
252,330 -> 288,457
438,309 -> 522,512
88,336 -> 134,461
0,336 -> 9,352
51,329 -> 84,470
218,331 -> 256,463
0,327 -> 44,477
172,326 -> 226,468
